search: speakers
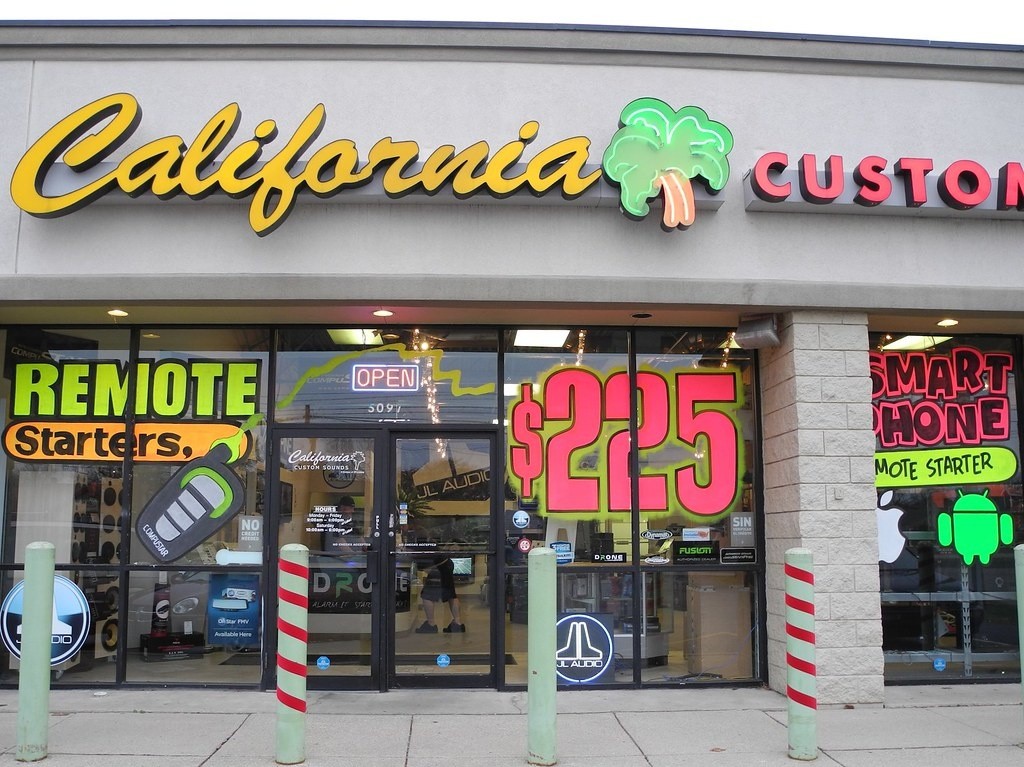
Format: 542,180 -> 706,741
734,318 -> 781,350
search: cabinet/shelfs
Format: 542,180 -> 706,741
558,571 -> 674,669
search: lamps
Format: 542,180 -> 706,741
732,315 -> 783,350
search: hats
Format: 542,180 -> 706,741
333,495 -> 356,507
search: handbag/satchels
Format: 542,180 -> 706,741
416,569 -> 429,584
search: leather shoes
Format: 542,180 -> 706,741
415,620 -> 438,634
442,619 -> 465,633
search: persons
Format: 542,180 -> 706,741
321,496 -> 367,562
394,516 -> 468,635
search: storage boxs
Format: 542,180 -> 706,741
139,631 -> 205,663
600,577 -> 615,598
623,623 -> 660,635
